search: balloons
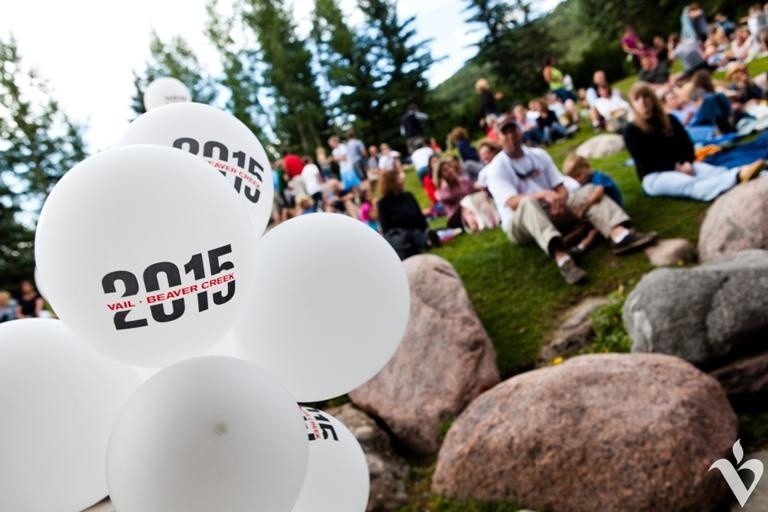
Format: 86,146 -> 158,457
1,76 -> 417,512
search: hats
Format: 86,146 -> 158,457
495,113 -> 518,131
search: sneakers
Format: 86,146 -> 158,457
614,232 -> 659,255
738,160 -> 764,181
559,260 -> 588,285
562,235 -> 586,256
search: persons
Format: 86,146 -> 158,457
264,2 -> 767,286
